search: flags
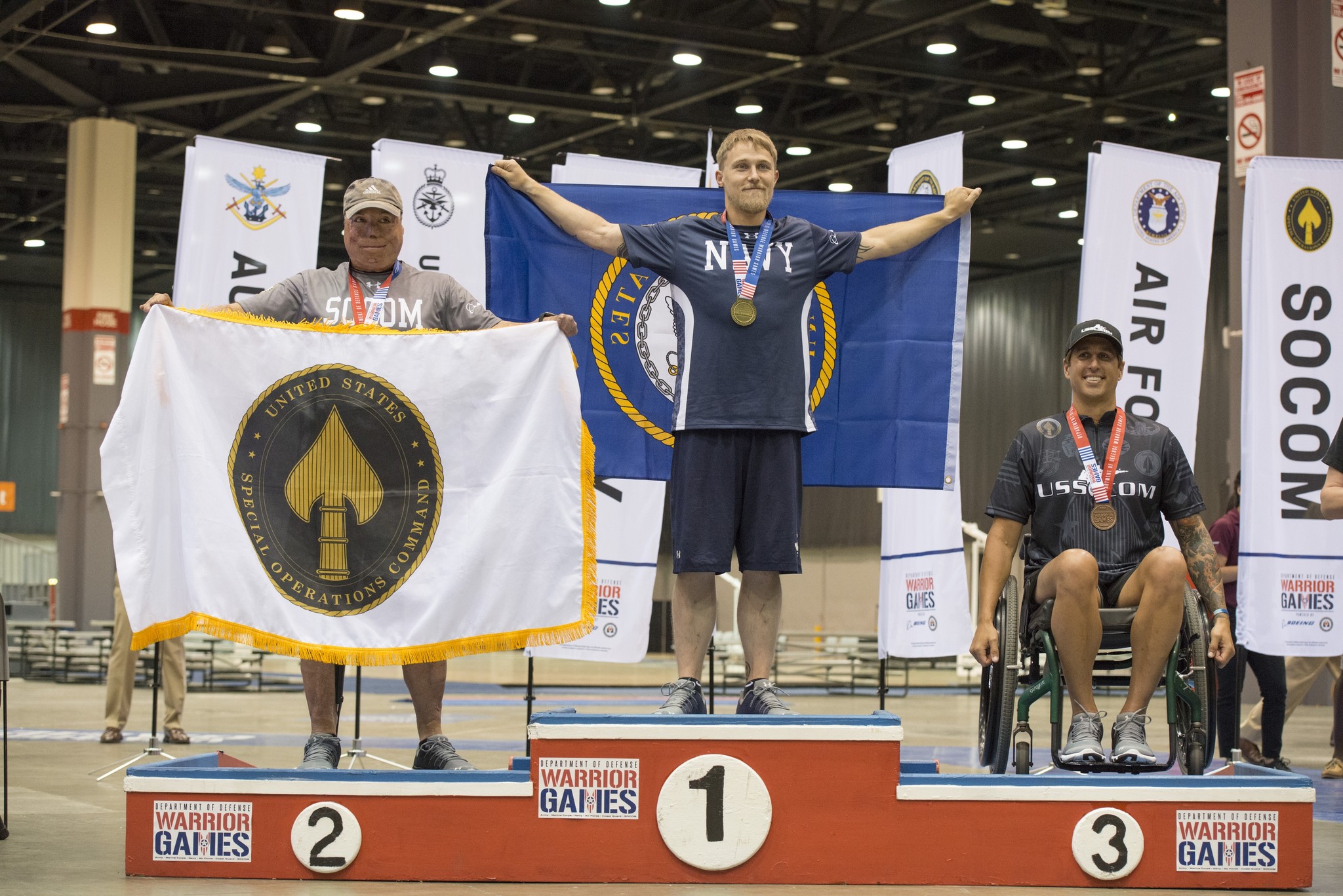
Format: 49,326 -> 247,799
98,305 -> 601,668
482,165 -> 972,492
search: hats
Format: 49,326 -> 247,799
343,177 -> 404,220
1065,319 -> 1124,356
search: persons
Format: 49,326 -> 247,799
139,177 -> 579,771
100,570 -> 193,744
491,129 -> 984,714
969,318 -> 1343,780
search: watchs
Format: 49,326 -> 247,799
539,312 -> 556,323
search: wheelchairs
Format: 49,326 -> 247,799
977,533 -> 1211,775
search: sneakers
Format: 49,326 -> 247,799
1061,699 -> 1108,761
163,728 -> 190,743
1263,759 -> 1292,772
1322,758 -> 1343,779
1109,706 -> 1156,763
650,677 -> 707,715
296,733 -> 341,769
101,727 -> 122,742
736,679 -> 799,715
412,734 -> 480,770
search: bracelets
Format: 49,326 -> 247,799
1212,609 -> 1228,619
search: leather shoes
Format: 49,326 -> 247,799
1239,737 -> 1261,766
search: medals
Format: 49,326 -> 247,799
731,297 -> 757,326
1090,502 -> 1117,530
